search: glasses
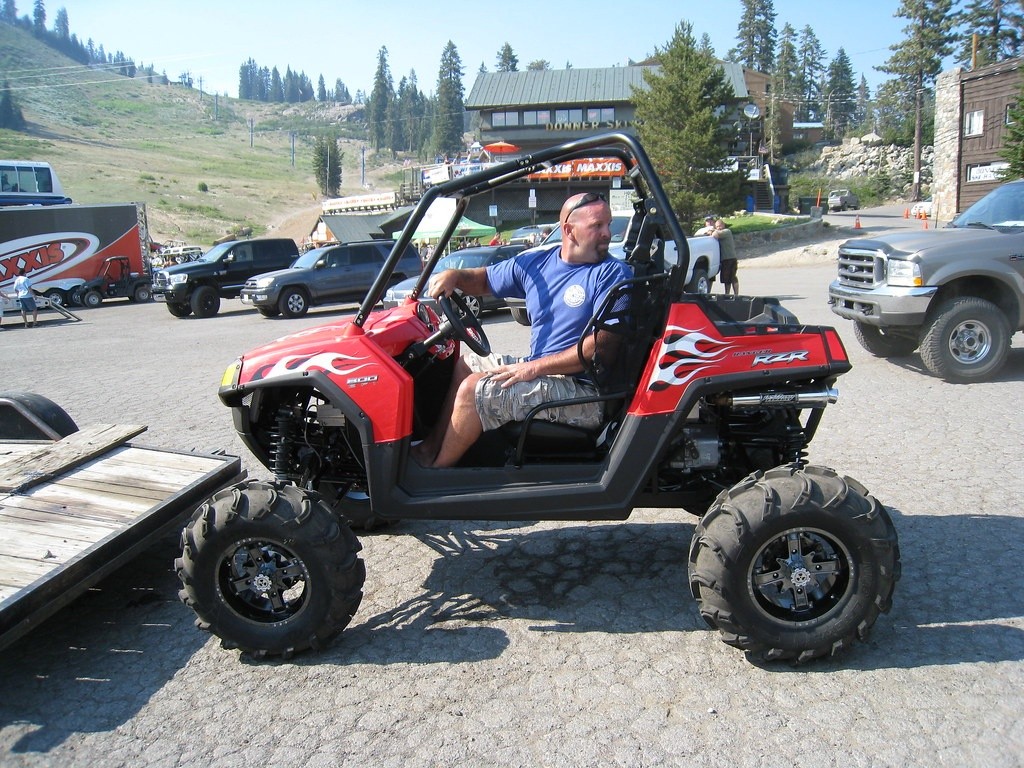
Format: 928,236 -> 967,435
565,193 -> 608,223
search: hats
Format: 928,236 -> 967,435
705,214 -> 714,220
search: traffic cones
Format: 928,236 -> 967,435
923,220 -> 928,230
921,210 -> 928,220
915,209 -> 920,219
904,208 -> 909,219
853,214 -> 862,229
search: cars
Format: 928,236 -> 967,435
910,196 -> 931,216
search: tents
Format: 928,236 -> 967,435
392,197 -> 496,256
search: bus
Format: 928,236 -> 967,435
0,158 -> 73,206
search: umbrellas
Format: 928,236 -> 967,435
481,141 -> 521,161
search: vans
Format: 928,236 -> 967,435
149,234 -> 203,271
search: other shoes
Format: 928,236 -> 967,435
25,324 -> 32,328
33,324 -> 41,327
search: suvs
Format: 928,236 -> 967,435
381,242 -> 531,319
240,239 -> 426,318
150,237 -> 300,319
827,189 -> 860,212
171,130 -> 903,668
828,179 -> 1024,384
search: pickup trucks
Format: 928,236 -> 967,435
508,208 -> 723,327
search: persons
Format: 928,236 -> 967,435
467,151 -> 472,164
415,243 -> 428,258
694,217 -> 739,294
489,233 -> 506,246
163,256 -> 178,268
0,269 -> 41,331
409,193 -> 636,467
444,155 -> 450,170
459,237 -> 481,249
453,155 -> 461,177
543,227 -> 552,237
